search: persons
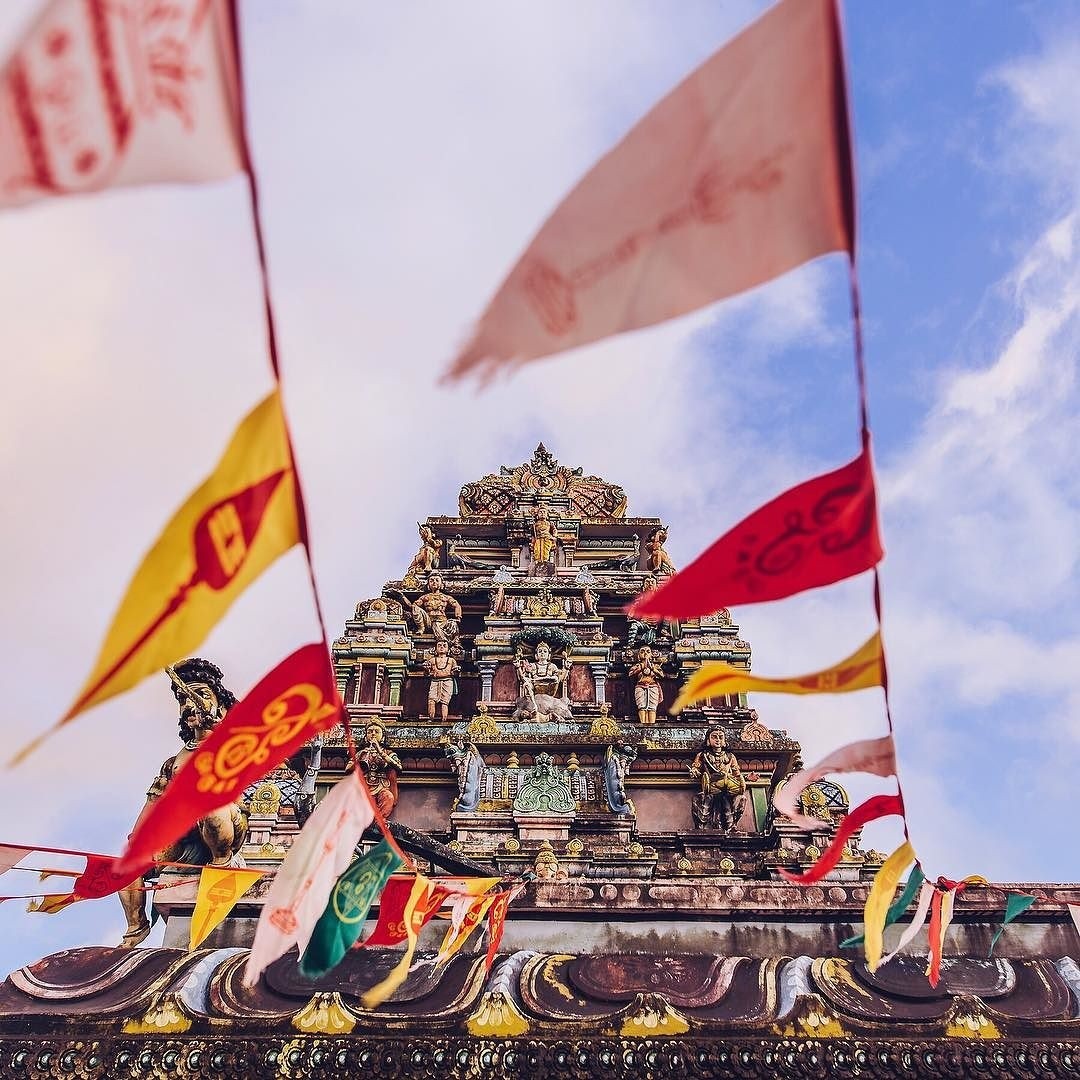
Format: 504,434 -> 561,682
513,640 -> 572,718
119,658 -> 248,949
629,645 -> 665,723
412,567 -> 463,638
413,522 -> 442,572
689,724 -> 761,831
644,524 -> 676,573
530,505 -> 559,564
423,640 -> 462,723
346,716 -> 402,830
626,573 -> 673,645
